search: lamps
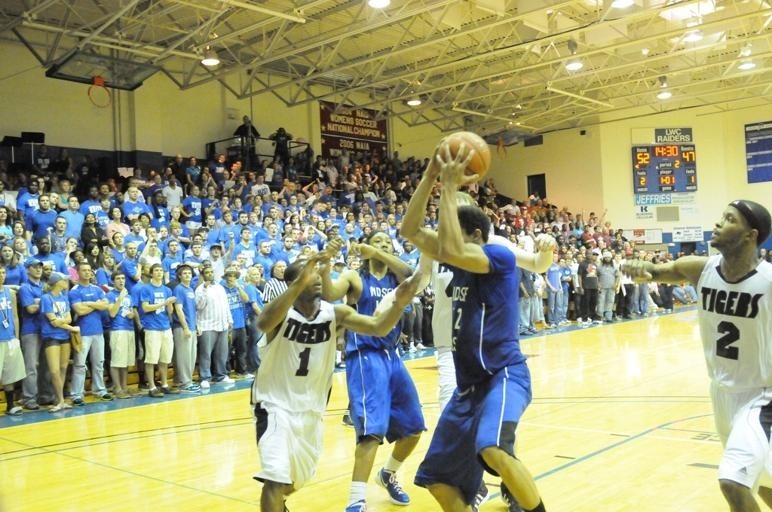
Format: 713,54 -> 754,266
201,46 -> 222,67
405,91 -> 422,107
565,56 -> 584,71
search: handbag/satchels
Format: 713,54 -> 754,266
70,329 -> 81,353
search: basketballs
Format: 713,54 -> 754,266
437,131 -> 493,184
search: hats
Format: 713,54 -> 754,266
48,272 -> 72,285
602,251 -> 612,258
220,266 -> 241,280
24,258 -> 43,268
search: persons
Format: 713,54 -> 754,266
251,136 -> 557,511
619,200 -> 772,512
1,118 -> 697,416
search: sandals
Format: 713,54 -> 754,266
335,360 -> 346,368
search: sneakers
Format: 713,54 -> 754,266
4,372 -> 255,418
375,466 -> 410,506
471,479 -> 490,510
500,480 -> 525,512
518,296 -> 708,336
345,499 -> 367,512
398,343 -> 427,355
